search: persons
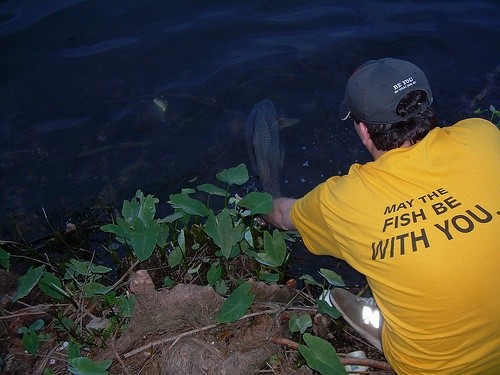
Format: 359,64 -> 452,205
258,58 -> 499,375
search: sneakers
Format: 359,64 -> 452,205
329,288 -> 384,352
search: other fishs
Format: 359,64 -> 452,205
245,98 -> 282,198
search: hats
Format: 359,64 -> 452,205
338,58 -> 432,124
